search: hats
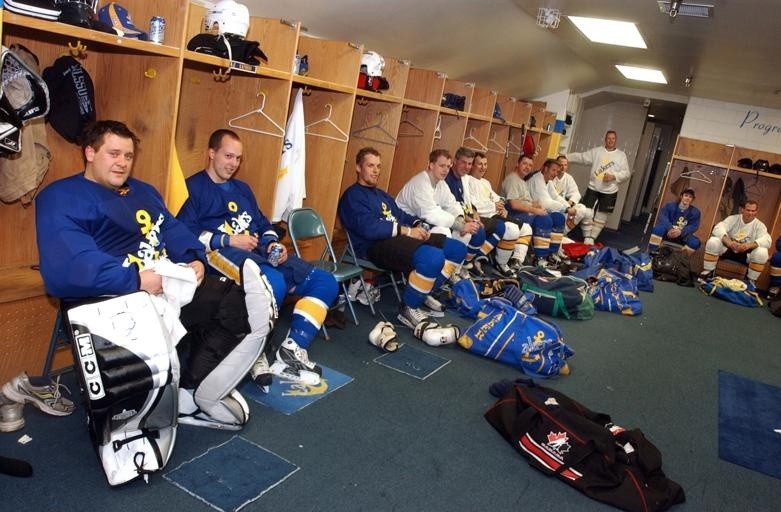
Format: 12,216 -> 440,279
98,2 -> 147,39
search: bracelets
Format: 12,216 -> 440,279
407,226 -> 412,237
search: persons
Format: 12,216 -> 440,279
766,236 -> 781,300
174,129 -> 340,396
647,188 -> 702,287
35,120 -> 280,431
337,147 -> 468,347
557,129 -> 631,247
395,148 -> 588,297
697,200 -> 773,299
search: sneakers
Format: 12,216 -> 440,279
742,278 -> 756,291
397,238 -> 594,331
249,352 -> 272,395
0,394 -> 25,432
270,338 -> 322,386
2,370 -> 75,417
698,269 -> 715,284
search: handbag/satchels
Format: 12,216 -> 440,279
574,248 -> 653,315
483,378 -> 686,510
651,246 -> 694,287
699,273 -> 764,307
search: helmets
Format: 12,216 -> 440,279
753,159 -> 769,173
361,50 -> 385,77
737,158 -> 753,168
770,164 -> 781,175
55,1 -> 97,28
205,0 -> 249,41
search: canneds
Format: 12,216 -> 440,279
546,123 -> 551,130
150,15 -> 165,44
294,55 -> 302,74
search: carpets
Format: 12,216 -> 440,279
717,368 -> 780,479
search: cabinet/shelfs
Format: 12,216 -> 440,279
648,135 -> 777,293
